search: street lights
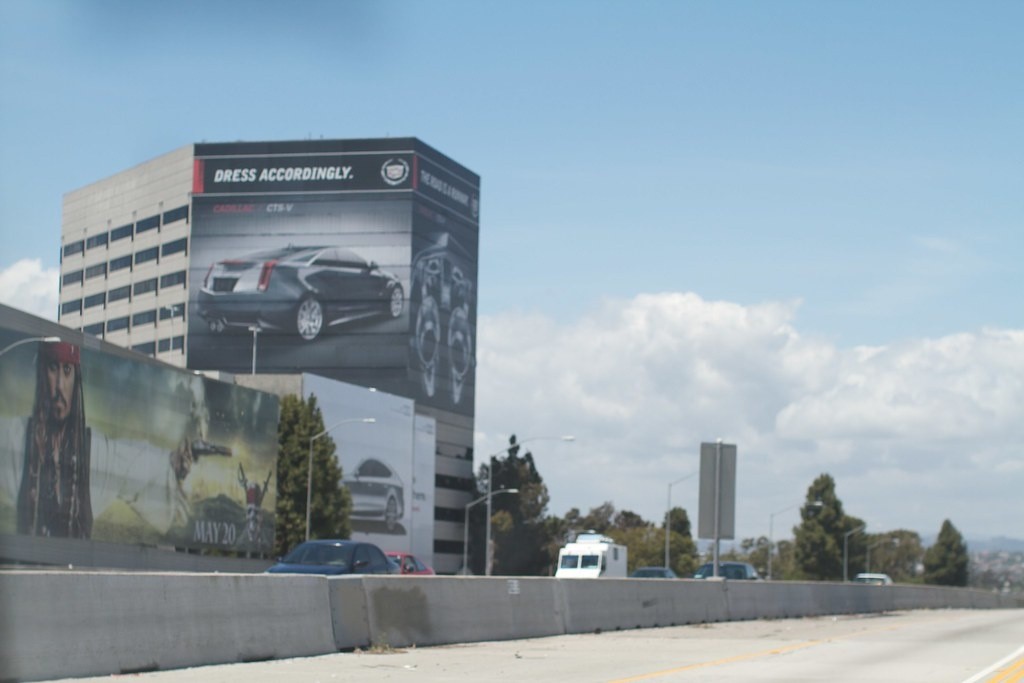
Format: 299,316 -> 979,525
766,500 -> 823,580
483,436 -> 579,580
304,415 -> 378,539
459,489 -> 521,574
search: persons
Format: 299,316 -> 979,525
15,338 -> 92,540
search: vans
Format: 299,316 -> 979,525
556,534 -> 627,583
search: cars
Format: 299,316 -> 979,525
631,567 -> 677,578
344,455 -> 406,533
198,241 -> 405,345
856,573 -> 896,586
265,539 -> 401,576
694,561 -> 757,580
357,551 -> 435,575
